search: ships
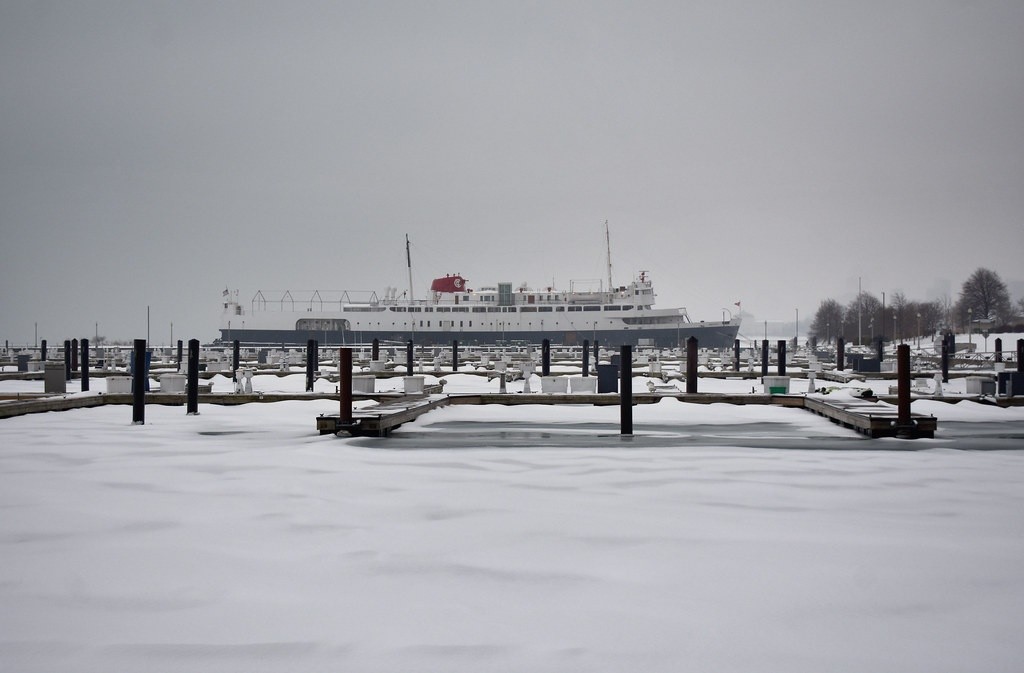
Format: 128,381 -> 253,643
219,218 -> 744,350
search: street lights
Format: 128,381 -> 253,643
762,305 -> 981,353
